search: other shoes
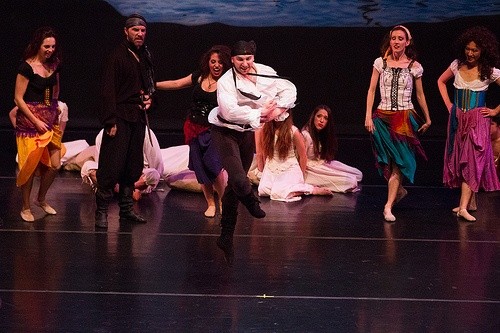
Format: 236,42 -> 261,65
20,197 -> 57,222
453,206 -> 478,221
383,188 -> 408,222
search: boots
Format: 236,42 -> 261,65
216,217 -> 237,267
235,188 -> 266,218
94,183 -> 113,228
117,184 -> 148,224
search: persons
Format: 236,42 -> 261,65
437,28 -> 500,225
207,36 -> 299,271
79,115 -> 166,204
297,106 -> 364,193
93,14 -> 156,229
154,44 -> 231,217
488,113 -> 500,183
15,26 -> 63,222
365,25 -> 433,222
12,100 -> 89,181
254,114 -> 333,202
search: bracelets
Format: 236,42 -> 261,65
425,123 -> 430,128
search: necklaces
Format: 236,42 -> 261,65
209,74 -> 218,89
252,65 -> 258,73
